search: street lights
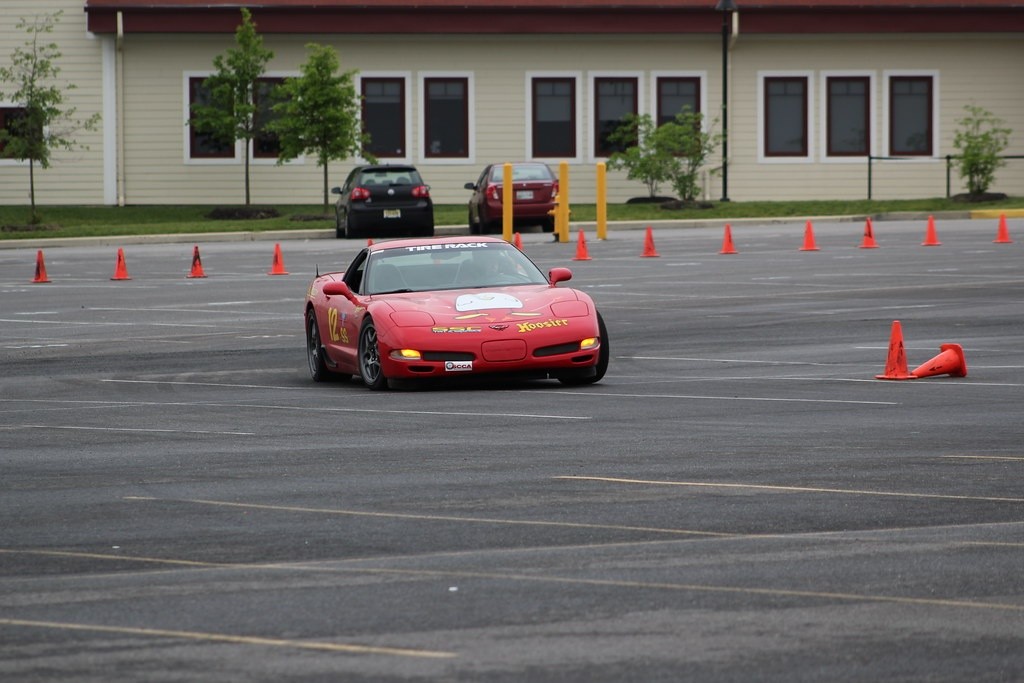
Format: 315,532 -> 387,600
715,0 -> 737,202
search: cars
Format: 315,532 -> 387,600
464,161 -> 560,234
330,165 -> 436,240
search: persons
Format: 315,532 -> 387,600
461,250 -> 524,285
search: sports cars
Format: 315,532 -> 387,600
305,236 -> 609,391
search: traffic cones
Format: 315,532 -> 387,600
569,227 -> 592,260
797,220 -> 819,251
875,320 -> 919,381
992,213 -> 1012,244
910,343 -> 967,379
641,226 -> 660,257
367,239 -> 374,247
109,246 -> 132,280
187,243 -> 207,278
269,242 -> 288,275
858,216 -> 880,248
920,215 -> 942,246
719,221 -> 739,254
514,231 -> 524,249
31,249 -> 51,283
